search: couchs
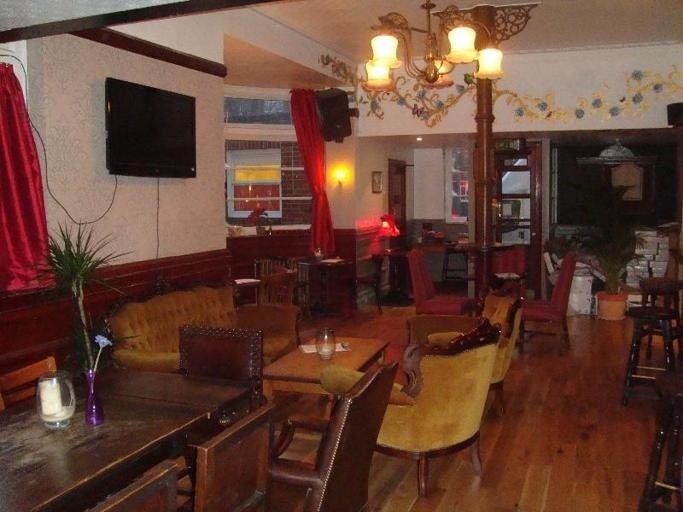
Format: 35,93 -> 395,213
109,286 -> 301,372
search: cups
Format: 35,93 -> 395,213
35,371 -> 76,430
315,328 -> 336,360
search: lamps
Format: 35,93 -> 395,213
377,214 -> 400,248
358,0 -> 506,93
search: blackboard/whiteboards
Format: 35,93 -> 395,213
552,143 -> 677,235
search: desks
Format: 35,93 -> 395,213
0,370 -> 259,512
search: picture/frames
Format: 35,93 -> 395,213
370,171 -> 383,193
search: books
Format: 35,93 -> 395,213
300,341 -> 350,354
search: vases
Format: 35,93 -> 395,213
84,369 -> 104,427
570,162 -> 644,321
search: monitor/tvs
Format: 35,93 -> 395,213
105,77 -> 197,179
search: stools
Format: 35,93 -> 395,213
621,306 -> 682,406
636,371 -> 683,512
638,277 -> 682,310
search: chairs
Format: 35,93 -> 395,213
518,257 -> 576,349
176,400 -> 274,511
268,360 -> 397,512
178,324 -> 264,409
421,290 -> 522,412
407,248 -> 474,315
322,318 -> 500,498
84,460 -> 178,510
0,354 -> 58,411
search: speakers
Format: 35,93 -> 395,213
667,103 -> 683,128
315,88 -> 352,143
